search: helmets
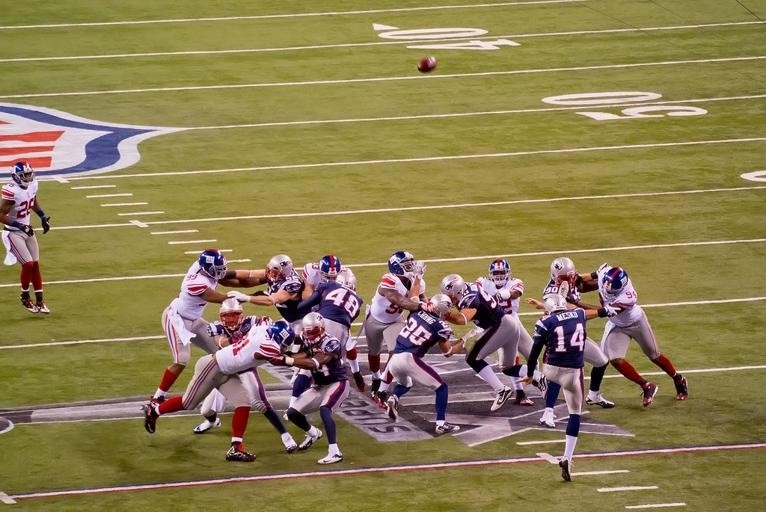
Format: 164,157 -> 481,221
199,249 -> 228,280
388,251 -> 415,280
544,294 -> 567,314
265,254 -> 294,282
603,267 -> 629,294
551,257 -> 578,289
488,259 -> 510,287
428,274 -> 466,321
10,160 -> 34,187
301,312 -> 326,344
320,254 -> 357,291
268,319 -> 295,353
219,299 -> 243,331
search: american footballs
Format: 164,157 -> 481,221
417,55 -> 436,73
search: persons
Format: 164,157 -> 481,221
143,249 -> 689,487
0,162 -> 50,314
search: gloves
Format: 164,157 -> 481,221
227,291 -> 251,303
19,223 -> 34,237
41,216 -> 51,234
460,328 -> 477,344
412,261 -> 427,281
604,302 -> 621,317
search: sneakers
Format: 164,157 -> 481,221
674,377 -> 688,400
352,370 -> 366,392
192,417 -> 221,434
640,381 -> 658,406
435,422 -> 461,434
585,393 -> 615,408
298,427 -> 323,450
140,395 -> 164,433
225,444 -> 256,462
18,293 -> 49,313
490,374 -> 548,412
559,455 -> 574,481
371,372 -> 399,420
317,449 -> 343,464
282,433 -> 298,454
539,413 -> 557,428
